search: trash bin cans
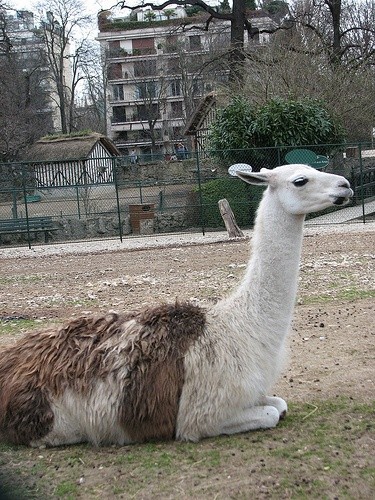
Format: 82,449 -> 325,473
129,203 -> 155,236
346,146 -> 360,160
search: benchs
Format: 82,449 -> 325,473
0,215 -> 59,243
